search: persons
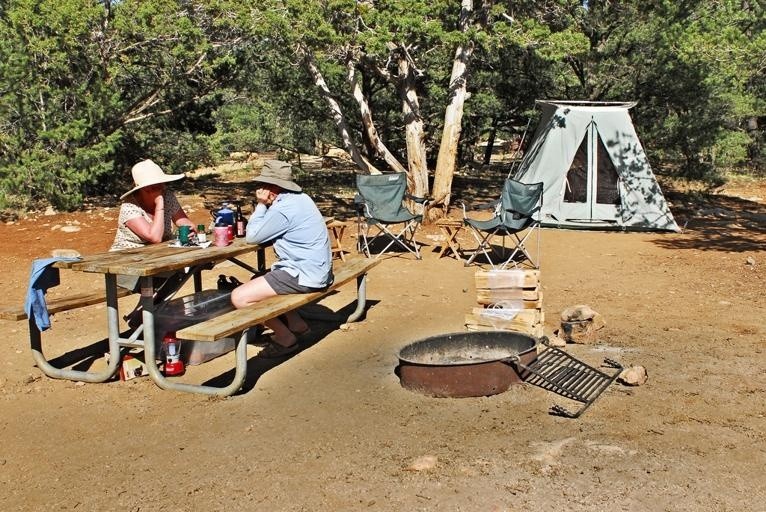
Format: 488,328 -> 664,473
229,160 -> 335,359
101,158 -> 205,328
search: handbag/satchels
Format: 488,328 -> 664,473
217,274 -> 244,290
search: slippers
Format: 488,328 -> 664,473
258,341 -> 299,358
291,327 -> 312,337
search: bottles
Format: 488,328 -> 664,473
235,203 -> 245,237
214,223 -> 229,247
196,224 -> 207,242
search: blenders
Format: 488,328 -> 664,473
162,336 -> 185,376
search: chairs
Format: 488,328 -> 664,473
352,172 -> 544,271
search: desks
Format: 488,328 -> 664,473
327,220 -> 347,259
33,215 -> 335,396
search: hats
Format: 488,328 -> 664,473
252,159 -> 303,192
119,159 -> 186,200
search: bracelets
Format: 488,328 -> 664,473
154,207 -> 165,212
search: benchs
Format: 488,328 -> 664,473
3,255 -> 211,396
176,259 -> 381,397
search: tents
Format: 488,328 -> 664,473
502,96 -> 683,234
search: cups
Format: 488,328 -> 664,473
215,209 -> 234,226
176,225 -> 191,243
